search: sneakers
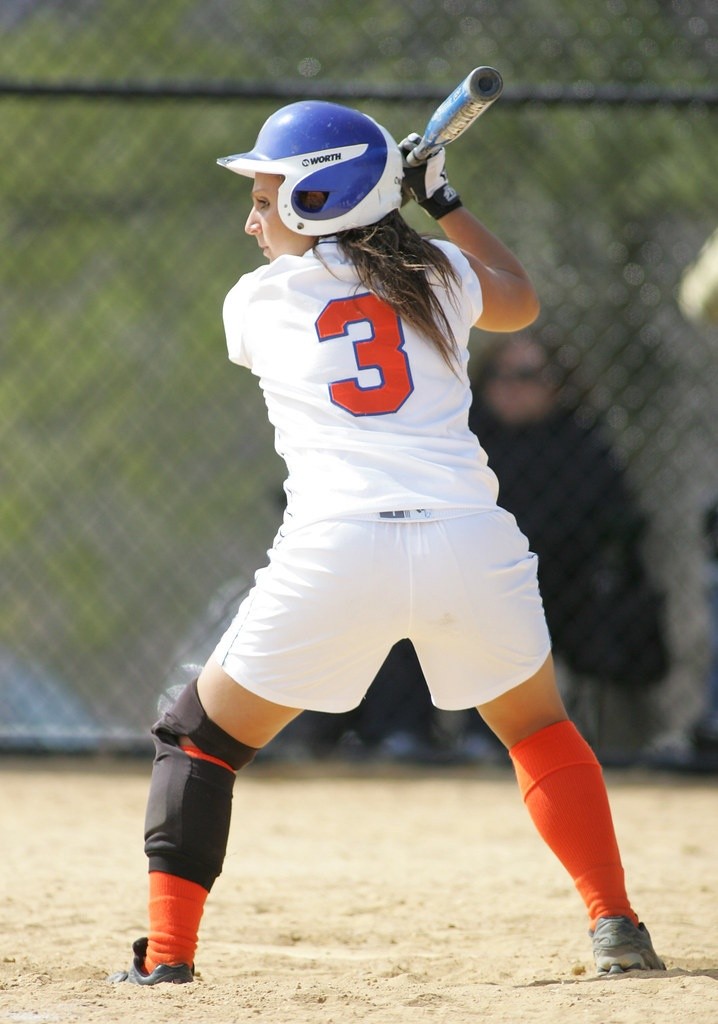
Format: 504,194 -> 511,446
587,917 -> 665,971
106,937 -> 193,985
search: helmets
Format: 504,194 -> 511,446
216,101 -> 403,237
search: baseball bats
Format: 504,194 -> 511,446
406,65 -> 504,168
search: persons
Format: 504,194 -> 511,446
109,99 -> 665,985
648,232 -> 718,774
336,329 -> 673,771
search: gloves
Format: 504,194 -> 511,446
398,134 -> 464,220
